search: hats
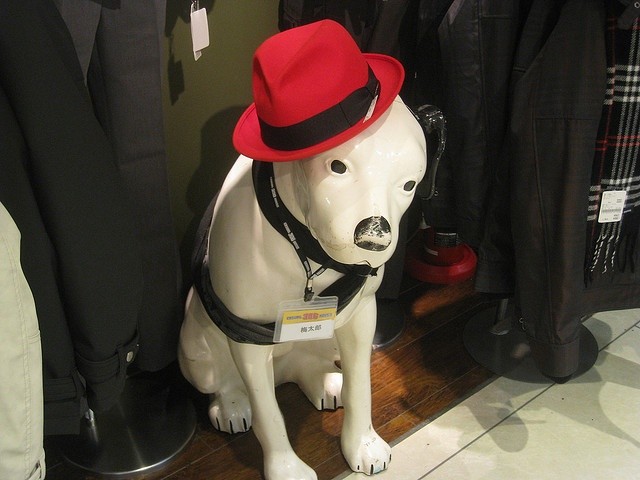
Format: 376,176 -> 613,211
231,20 -> 405,162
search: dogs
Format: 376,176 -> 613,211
178,93 -> 427,480
